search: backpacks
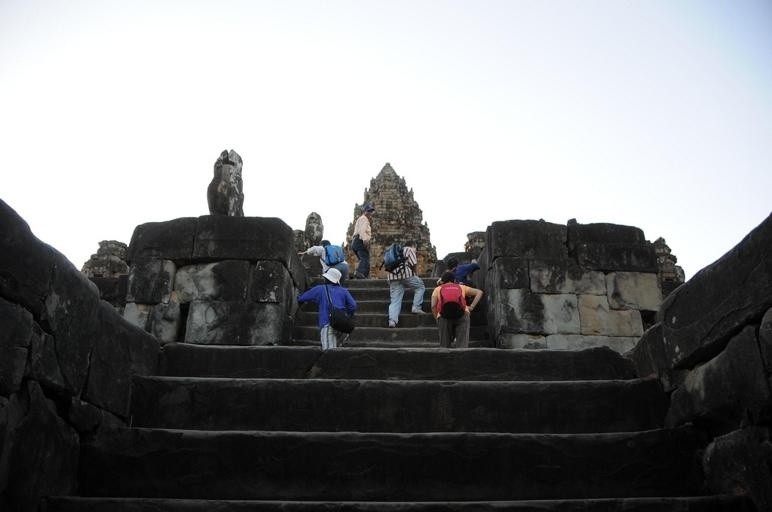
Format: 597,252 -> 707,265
383,242 -> 404,272
320,245 -> 345,266
438,285 -> 465,320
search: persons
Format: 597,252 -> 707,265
351,204 -> 375,279
297,239 -> 349,286
297,267 -> 357,350
387,240 -> 426,327
447,257 -> 481,286
431,270 -> 483,348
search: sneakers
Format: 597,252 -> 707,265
412,307 -> 425,314
389,320 -> 396,327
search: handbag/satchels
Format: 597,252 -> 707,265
328,307 -> 354,335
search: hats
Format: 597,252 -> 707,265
322,268 -> 342,285
365,205 -> 375,211
